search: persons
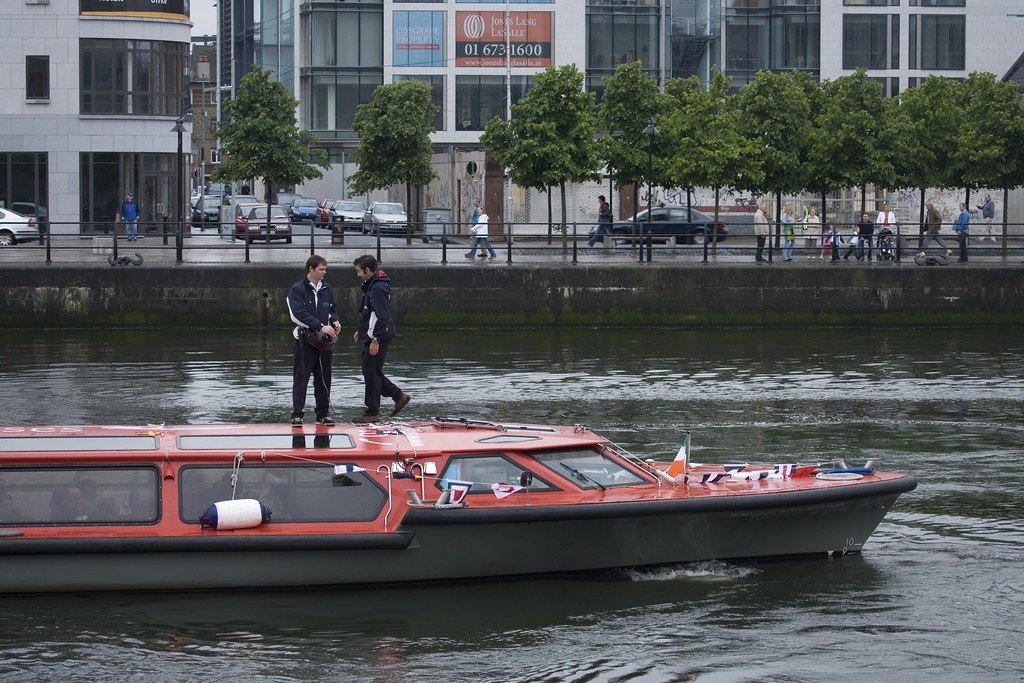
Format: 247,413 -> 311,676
956,202 -> 971,263
877,202 -> 897,233
782,203 -> 797,261
465,198 -> 496,260
857,215 -> 874,260
286,255 -> 342,427
585,195 -> 614,247
843,226 -> 866,262
121,192 -> 141,241
754,203 -> 771,261
818,225 -> 845,263
881,235 -> 894,255
924,200 -> 953,257
975,194 -> 997,244
352,255 -> 411,425
801,207 -> 820,259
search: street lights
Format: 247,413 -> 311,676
642,117 -> 658,262
169,90 -> 196,264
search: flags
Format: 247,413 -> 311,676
334,464 -> 523,504
666,438 -> 688,478
699,465 -> 819,483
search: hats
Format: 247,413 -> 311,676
128,192 -> 133,196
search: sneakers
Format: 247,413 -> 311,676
315,416 -> 335,425
291,417 -> 303,426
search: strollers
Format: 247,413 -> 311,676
876,228 -> 895,261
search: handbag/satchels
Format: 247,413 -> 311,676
923,225 -> 929,231
784,224 -> 797,240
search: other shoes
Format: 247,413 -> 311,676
760,258 -> 767,261
812,256 -> 815,258
843,258 -> 849,262
127,239 -> 132,241
807,257 -> 810,259
828,255 -> 832,259
859,256 -> 864,262
488,255 -> 496,260
465,255 -> 473,259
478,254 -> 487,257
916,253 -> 926,258
352,416 -> 379,423
585,241 -> 593,247
134,239 -> 136,241
390,393 -> 411,417
819,254 -> 824,260
945,250 -> 952,256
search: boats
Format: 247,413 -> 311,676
0,413 -> 919,595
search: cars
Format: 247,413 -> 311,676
0,207 -> 41,246
286,196 -> 320,225
11,201 -> 46,232
361,201 -> 415,237
235,202 -> 270,238
588,205 -> 730,245
313,199 -> 347,229
190,184 -> 260,229
274,192 -> 305,216
242,205 -> 294,245
327,200 -> 367,232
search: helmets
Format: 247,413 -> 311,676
304,328 -> 338,351
